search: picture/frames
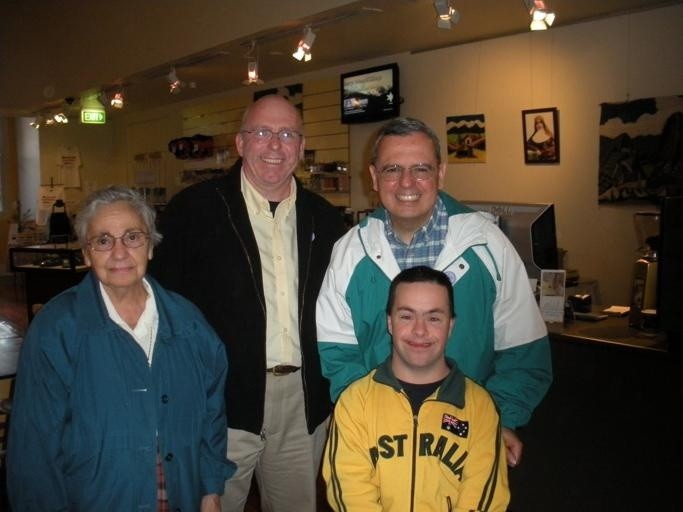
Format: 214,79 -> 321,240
597,97 -> 683,205
444,114 -> 488,165
521,107 -> 560,166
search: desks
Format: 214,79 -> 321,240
1,321 -> 25,459
534,302 -> 682,448
7,260 -> 92,324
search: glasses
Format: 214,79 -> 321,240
378,162 -> 434,185
236,125 -> 305,143
92,227 -> 152,249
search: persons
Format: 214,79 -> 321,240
526,116 -> 552,158
5,190 -> 237,511
146,96 -> 347,511
320,267 -> 510,511
314,118 -> 553,470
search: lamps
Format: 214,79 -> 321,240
108,84 -> 127,111
291,22 -> 318,63
27,109 -> 70,130
155,60 -> 188,97
523,0 -> 558,31
432,0 -> 461,30
241,40 -> 267,86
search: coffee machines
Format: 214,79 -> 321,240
630,208 -> 665,334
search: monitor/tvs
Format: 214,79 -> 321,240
461,202 -> 560,283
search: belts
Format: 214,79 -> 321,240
267,362 -> 295,382
